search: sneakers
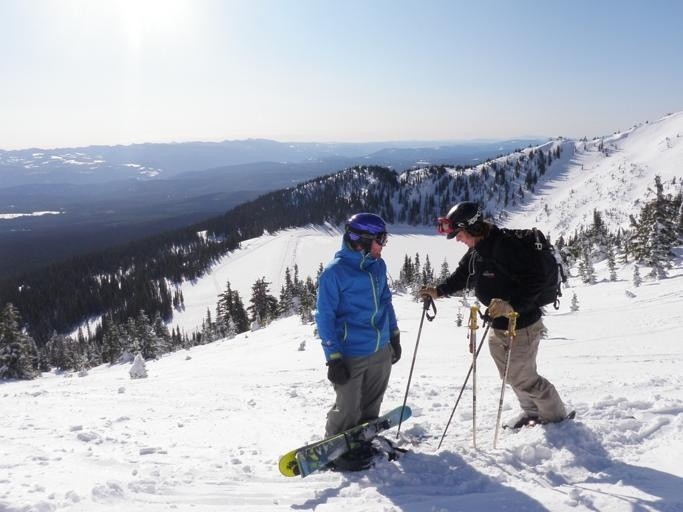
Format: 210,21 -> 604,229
509,412 -> 565,429
333,435 -> 397,470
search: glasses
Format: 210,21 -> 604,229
345,224 -> 388,246
436,218 -> 465,234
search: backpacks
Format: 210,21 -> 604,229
496,227 -> 558,308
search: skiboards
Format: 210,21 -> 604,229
502,410 -> 575,434
279,406 -> 411,478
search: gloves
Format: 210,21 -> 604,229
390,342 -> 402,364
326,360 -> 351,384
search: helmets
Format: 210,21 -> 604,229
446,201 -> 485,240
345,213 -> 387,240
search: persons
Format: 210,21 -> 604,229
314,213 -> 402,471
417,201 -> 567,430
